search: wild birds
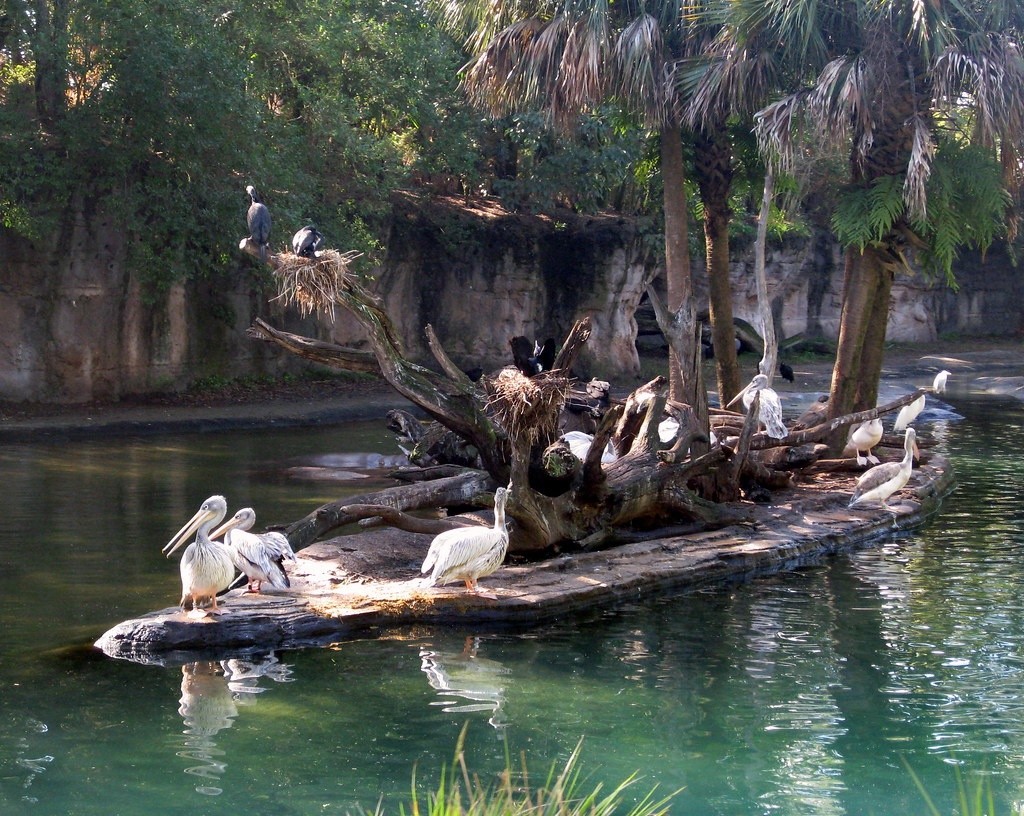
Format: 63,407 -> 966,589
419,636 -> 516,729
292,223 -> 324,262
243,184 -> 272,287
162,495 -> 297,620
178,650 -> 297,744
421,488 -> 513,593
468,367 -> 483,382
511,333 -> 556,380
726,374 -> 790,440
586,376 -> 611,402
842,419 -> 920,512
780,361 -> 795,383
932,370 -> 951,396
